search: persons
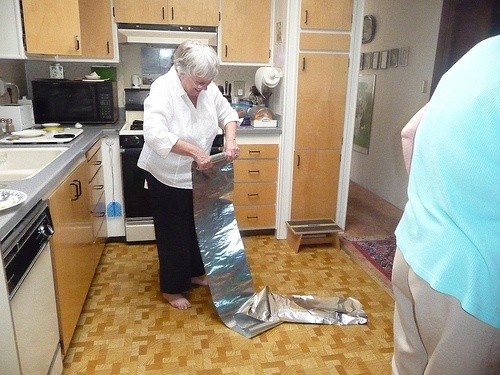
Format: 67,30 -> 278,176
136,39 -> 240,310
391,36 -> 500,375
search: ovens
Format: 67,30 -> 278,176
120,147 -> 224,242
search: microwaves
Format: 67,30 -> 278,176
32,78 -> 119,124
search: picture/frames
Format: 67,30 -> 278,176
360,53 -> 364,70
398,46 -> 409,67
389,48 -> 399,68
362,14 -> 376,44
276,21 -> 283,43
380,50 -> 389,70
371,52 -> 380,70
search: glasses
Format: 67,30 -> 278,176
187,73 -> 213,92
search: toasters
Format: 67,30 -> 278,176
0,104 -> 35,131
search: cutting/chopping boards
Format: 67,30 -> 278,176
0,128 -> 84,144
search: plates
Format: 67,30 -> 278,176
0,189 -> 27,211
85,76 -> 101,80
11,130 -> 46,137
42,123 -> 61,128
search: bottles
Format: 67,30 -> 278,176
6,118 -> 15,135
0,118 -> 6,137
49,63 -> 64,79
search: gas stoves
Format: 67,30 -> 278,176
119,86 -> 223,147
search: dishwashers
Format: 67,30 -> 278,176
0,198 -> 65,375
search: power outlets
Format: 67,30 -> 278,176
234,81 -> 245,97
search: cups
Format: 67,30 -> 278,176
131,74 -> 142,87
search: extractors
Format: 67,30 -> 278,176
117,23 -> 218,47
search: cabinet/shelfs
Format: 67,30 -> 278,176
289,0 -> 354,221
113,0 -> 218,27
217,0 -> 274,67
223,133 -> 279,231
41,155 -> 99,360
0,0 -> 82,63
76,0 -> 120,64
85,138 -> 107,263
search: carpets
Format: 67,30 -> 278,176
340,233 -> 397,300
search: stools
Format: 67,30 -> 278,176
285,218 -> 343,254
